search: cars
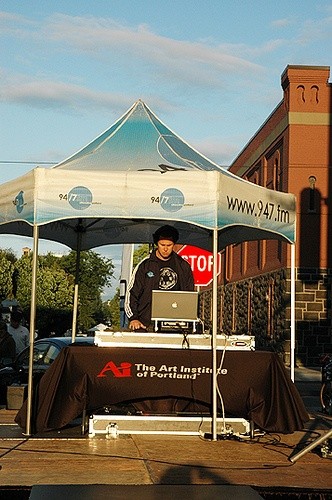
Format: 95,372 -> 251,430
11,337 -> 95,386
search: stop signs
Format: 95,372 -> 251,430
176,244 -> 222,287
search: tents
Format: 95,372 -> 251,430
0,99 -> 296,441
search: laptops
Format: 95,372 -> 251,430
152,290 -> 198,320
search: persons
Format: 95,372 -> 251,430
124,226 -> 195,329
0,314 -> 31,368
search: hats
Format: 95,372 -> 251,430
152,225 -> 179,246
